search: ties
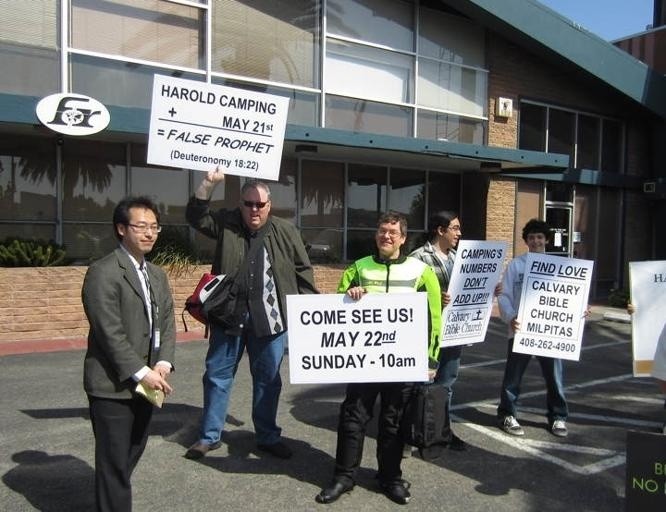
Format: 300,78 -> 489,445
140,266 -> 154,339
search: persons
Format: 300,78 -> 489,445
80,193 -> 177,511
625,298 -> 636,316
650,322 -> 666,393
404,212 -> 504,465
499,218 -> 592,441
313,209 -> 444,504
185,166 -> 320,464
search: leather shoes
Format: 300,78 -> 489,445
377,479 -> 410,504
255,444 -> 294,458
317,478 -> 355,502
185,440 -> 220,458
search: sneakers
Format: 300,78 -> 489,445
549,419 -> 567,437
496,415 -> 523,435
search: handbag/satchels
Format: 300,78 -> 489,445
185,274 -> 249,329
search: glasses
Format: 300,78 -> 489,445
447,226 -> 459,233
377,229 -> 401,237
121,222 -> 162,234
239,199 -> 269,209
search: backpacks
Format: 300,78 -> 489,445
405,382 -> 452,460
